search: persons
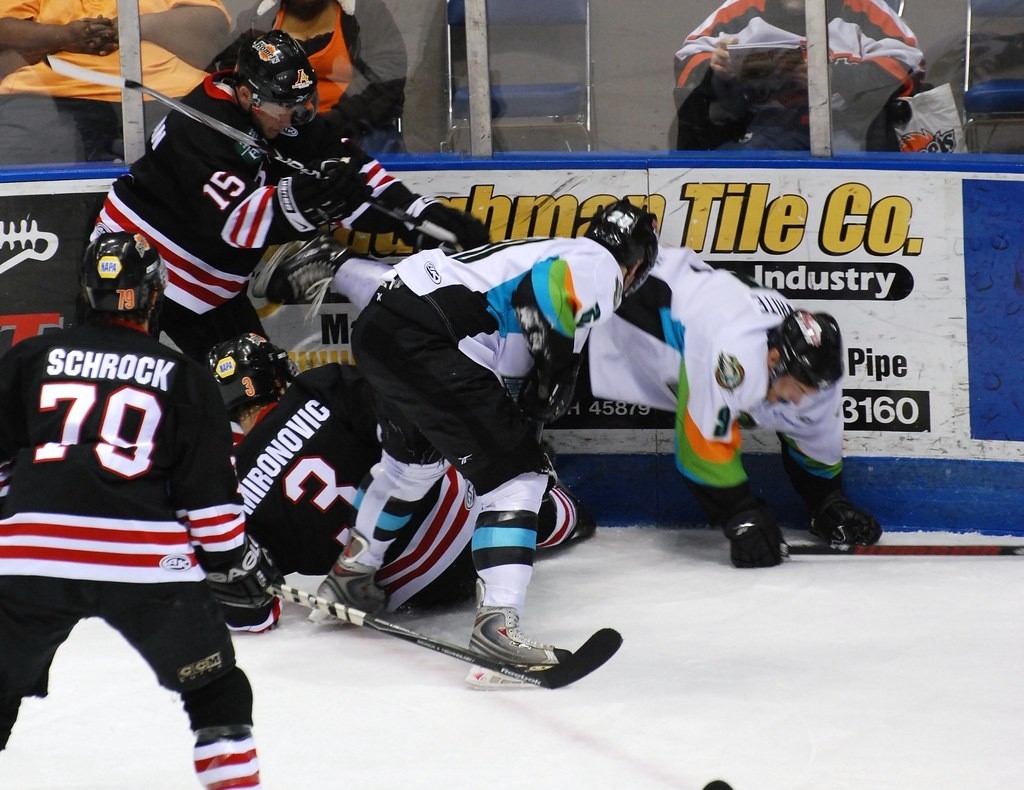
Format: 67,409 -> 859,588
207,331 -> 596,633
307,200 -> 658,677
229,0 -> 408,154
251,230 -> 883,569
0,0 -> 234,166
0,231 -> 286,790
673,0 -> 926,151
80,30 -> 491,446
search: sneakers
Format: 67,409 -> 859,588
253,238 -> 353,305
307,527 -> 386,622
466,577 -> 573,690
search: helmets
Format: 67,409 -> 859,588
584,198 -> 660,298
79,231 -> 162,315
777,310 -> 843,389
209,332 -> 289,410
238,29 -> 318,104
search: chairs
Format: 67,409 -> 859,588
962,0 -> 1024,150
444,0 -> 591,152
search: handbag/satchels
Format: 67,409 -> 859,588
894,82 -> 970,153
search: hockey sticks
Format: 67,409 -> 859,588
779,541 -> 1023,557
535,362 -> 553,444
38,53 -> 459,245
266,585 -> 627,691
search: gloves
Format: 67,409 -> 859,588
193,535 -> 286,635
271,162 -> 374,243
723,503 -> 789,569
808,493 -> 883,546
398,192 -> 491,254
517,329 -> 585,422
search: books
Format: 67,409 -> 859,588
725,40 -> 806,95
